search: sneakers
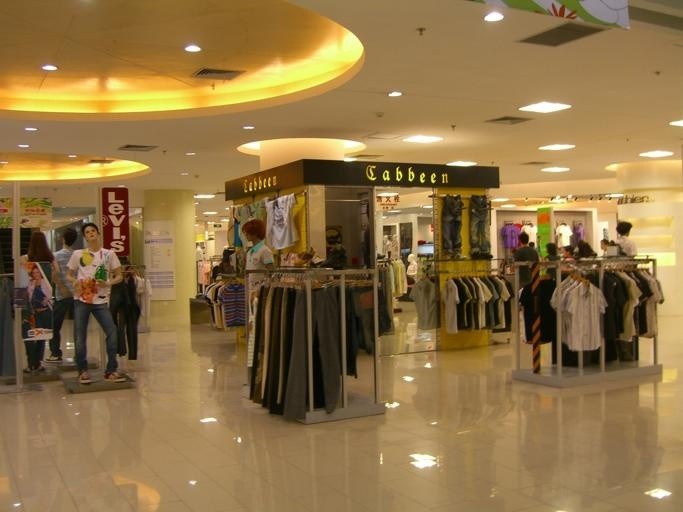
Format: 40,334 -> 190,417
444,252 -> 493,261
23,356 -> 62,373
78,371 -> 91,384
102,371 -> 126,383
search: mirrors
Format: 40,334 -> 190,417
374,186 -> 435,355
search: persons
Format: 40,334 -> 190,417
559,245 -> 576,274
576,240 -> 597,258
66,222 -> 128,383
405,253 -> 417,276
512,232 -> 539,289
26,266 -> 52,329
20,231 -> 62,374
44,227 -> 78,362
614,221 -> 638,269
241,219 -> 273,289
544,243 -> 560,280
599,239 -> 609,255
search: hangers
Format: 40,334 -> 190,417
261,271 -> 374,291
376,258 -> 405,269
444,268 -> 503,281
214,275 -> 242,286
416,269 -> 435,284
118,265 -> 147,280
533,258 -> 655,289
